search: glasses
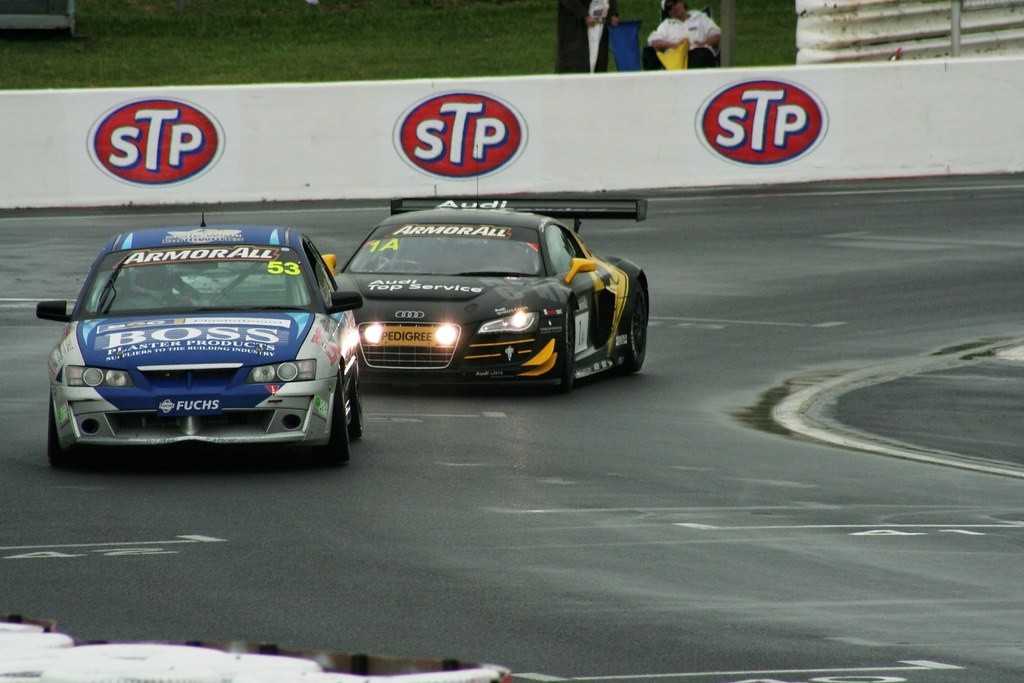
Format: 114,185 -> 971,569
666,0 -> 678,13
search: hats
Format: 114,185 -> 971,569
661,0 -> 666,11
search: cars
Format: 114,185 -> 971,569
34,228 -> 364,472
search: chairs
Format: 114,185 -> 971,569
470,240 -> 535,274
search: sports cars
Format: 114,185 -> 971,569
333,197 -> 649,394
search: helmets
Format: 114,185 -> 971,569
128,262 -> 173,298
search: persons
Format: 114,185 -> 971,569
641,0 -> 721,71
119,265 -> 210,310
555,0 -> 619,74
